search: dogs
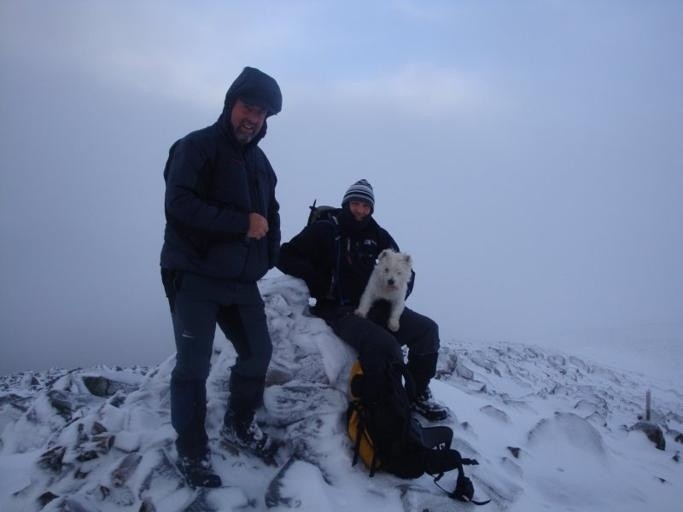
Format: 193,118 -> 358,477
354,247 -> 413,333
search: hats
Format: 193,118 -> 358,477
341,179 -> 375,212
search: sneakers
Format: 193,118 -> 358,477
409,386 -> 449,421
218,406 -> 285,458
176,447 -> 222,487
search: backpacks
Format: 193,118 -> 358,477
345,355 -> 461,480
305,200 -> 346,316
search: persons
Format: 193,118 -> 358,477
276,179 -> 449,420
161,67 -> 281,486
